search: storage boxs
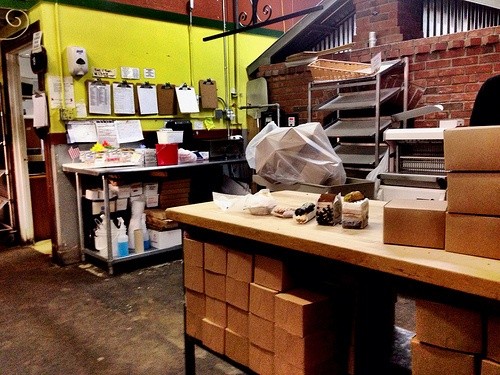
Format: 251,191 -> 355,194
183,230 -> 500,375
382,126 -> 500,262
84,182 -> 160,215
148,229 -> 182,249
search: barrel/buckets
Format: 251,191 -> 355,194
156,143 -> 178,166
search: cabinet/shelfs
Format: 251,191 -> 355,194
308,56 -> 409,172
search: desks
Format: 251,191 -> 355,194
165,190 -> 500,374
63,155 -> 247,276
